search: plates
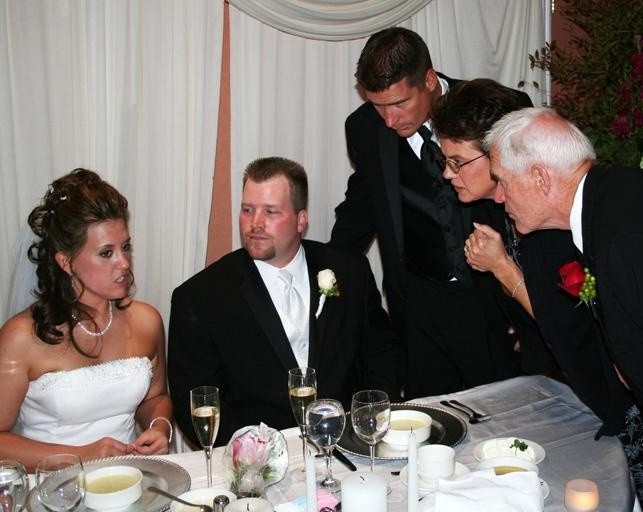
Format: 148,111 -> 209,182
39,455 -> 190,512
166,487 -> 236,511
539,475 -> 549,501
338,401 -> 465,459
399,461 -> 470,492
470,437 -> 545,465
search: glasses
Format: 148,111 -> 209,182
434,151 -> 489,174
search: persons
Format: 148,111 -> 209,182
481,106 -> 643,511
428,78 -> 615,436
326,26 -> 534,401
167,157 -> 401,451
0,168 -> 174,474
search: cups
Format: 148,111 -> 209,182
562,479 -> 599,511
414,444 -> 455,482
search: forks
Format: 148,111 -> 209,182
449,400 -> 489,417
440,399 -> 490,425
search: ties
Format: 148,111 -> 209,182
277,270 -> 309,375
416,126 -> 446,179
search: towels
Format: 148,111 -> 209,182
440,465 -> 543,509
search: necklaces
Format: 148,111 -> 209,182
72,300 -> 113,336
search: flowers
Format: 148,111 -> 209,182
556,261 -> 596,319
515,1 -> 642,169
312,267 -> 342,318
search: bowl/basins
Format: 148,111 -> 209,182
81,464 -> 141,511
376,407 -> 430,449
476,456 -> 540,476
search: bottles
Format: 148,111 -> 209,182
213,495 -> 228,512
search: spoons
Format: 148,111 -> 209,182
146,483 -> 211,511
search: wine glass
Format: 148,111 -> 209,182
349,388 -> 391,469
188,385 -> 222,487
34,453 -> 85,511
0,459 -> 27,512
302,397 -> 346,490
287,367 -> 318,477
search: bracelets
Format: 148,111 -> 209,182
511,278 -> 524,298
149,416 -> 173,444
